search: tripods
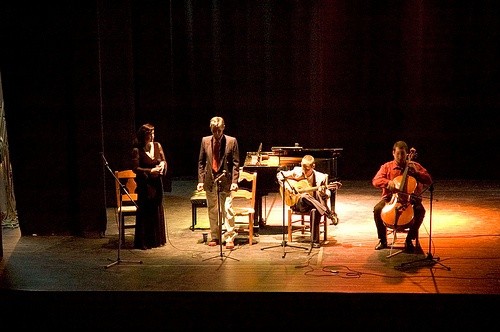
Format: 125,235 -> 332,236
201,174 -> 241,262
102,163 -> 142,269
260,171 -> 308,259
398,181 -> 450,271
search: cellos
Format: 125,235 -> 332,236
380,148 -> 418,257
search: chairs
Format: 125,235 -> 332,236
288,174 -> 329,241
225,171 -> 257,246
114,169 -> 138,239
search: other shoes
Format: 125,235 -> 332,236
405,240 -> 415,250
209,239 -> 219,246
226,242 -> 234,248
331,212 -> 338,225
312,242 -> 320,248
375,240 -> 387,250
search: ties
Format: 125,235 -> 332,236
212,140 -> 220,172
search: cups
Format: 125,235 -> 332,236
202,232 -> 208,242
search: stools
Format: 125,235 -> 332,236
190,188 -> 210,232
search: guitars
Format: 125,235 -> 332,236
280,179 -> 342,207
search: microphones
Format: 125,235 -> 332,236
99,152 -> 107,165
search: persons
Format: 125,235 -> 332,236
277,155 -> 337,248
372,141 -> 433,253
197,117 -> 240,249
130,124 -> 168,249
